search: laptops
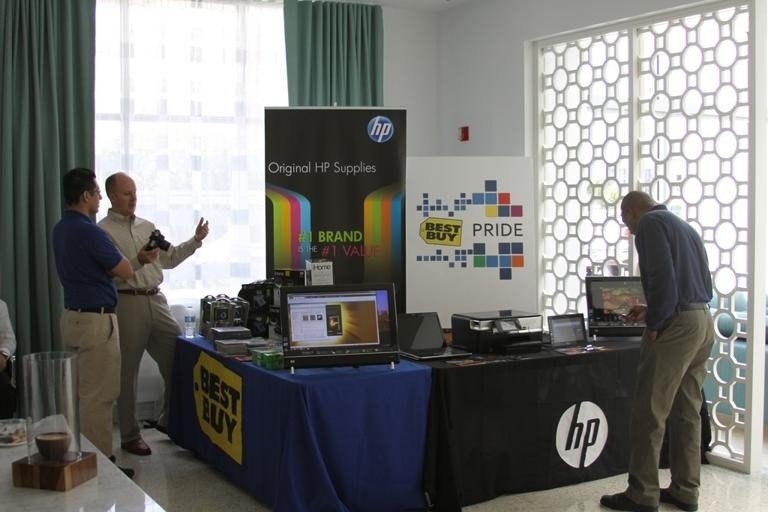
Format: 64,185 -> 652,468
397,313 -> 473,361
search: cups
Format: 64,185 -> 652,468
22,351 -> 82,465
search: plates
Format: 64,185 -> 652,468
0,416 -> 30,446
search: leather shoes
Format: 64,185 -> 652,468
600,492 -> 659,512
120,436 -> 151,455
155,424 -> 195,451
660,487 -> 698,511
118,465 -> 135,479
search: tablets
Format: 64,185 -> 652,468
548,313 -> 587,348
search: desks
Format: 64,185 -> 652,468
1,407 -> 168,512
168,327 -> 434,512
420,330 -> 712,511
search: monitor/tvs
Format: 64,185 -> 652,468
280,283 -> 400,375
585,276 -> 649,342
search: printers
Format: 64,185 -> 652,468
451,309 -> 543,355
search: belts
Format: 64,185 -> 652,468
117,286 -> 159,295
63,305 -> 116,313
676,302 -> 709,312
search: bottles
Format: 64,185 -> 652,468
184,305 -> 197,339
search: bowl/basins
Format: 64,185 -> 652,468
34,431 -> 73,460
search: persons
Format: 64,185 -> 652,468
0,300 -> 18,420
600,190 -> 717,512
96,172 -> 209,456
53,167 -> 135,478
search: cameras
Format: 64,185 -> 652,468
145,229 -> 170,252
